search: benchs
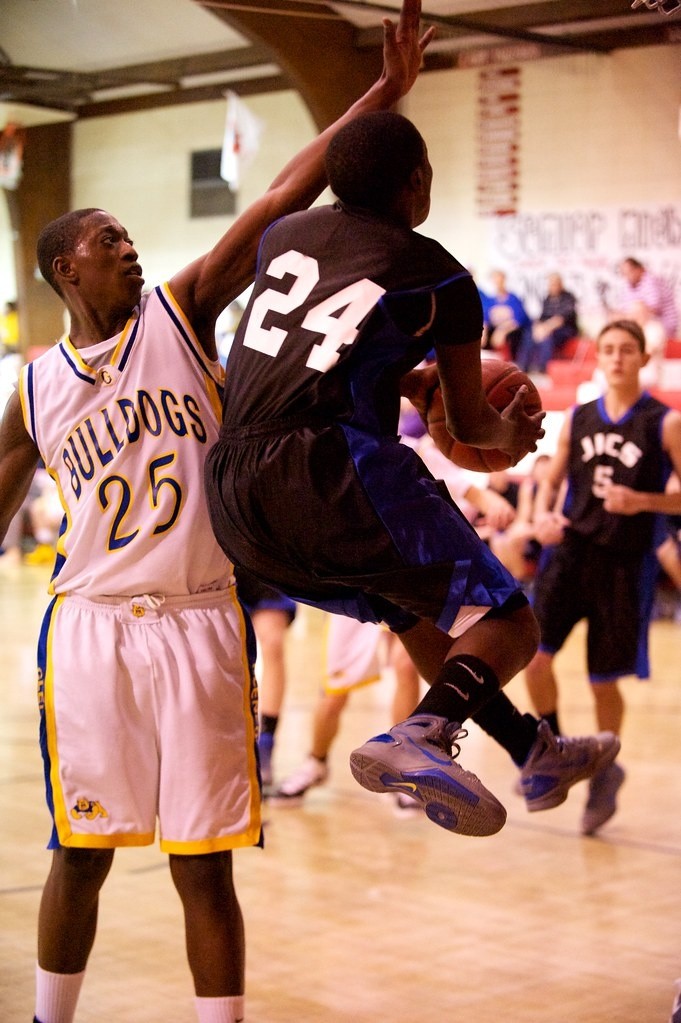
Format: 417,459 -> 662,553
499,336 -> 681,414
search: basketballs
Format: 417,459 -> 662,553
428,356 -> 542,477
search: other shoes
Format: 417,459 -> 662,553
276,765 -> 329,797
258,735 -> 274,785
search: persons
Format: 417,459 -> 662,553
201,114 -> 620,837
0,256 -> 681,835
0,2 -> 434,1022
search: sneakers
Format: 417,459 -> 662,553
520,712 -> 621,812
580,761 -> 624,834
350,714 -> 508,837
